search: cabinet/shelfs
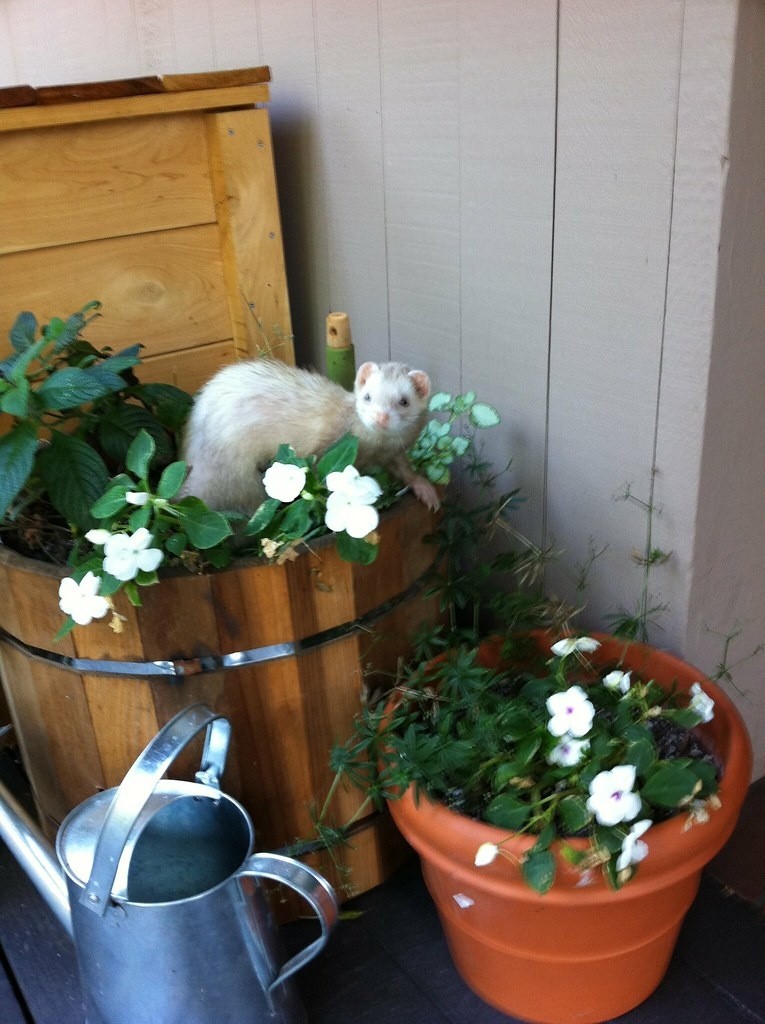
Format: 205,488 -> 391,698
0,63 -> 300,443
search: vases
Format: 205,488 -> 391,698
0,472 -> 452,920
377,626 -> 755,1024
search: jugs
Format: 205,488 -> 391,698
0,703 -> 339,1024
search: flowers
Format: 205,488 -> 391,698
0,302 -> 764,923
